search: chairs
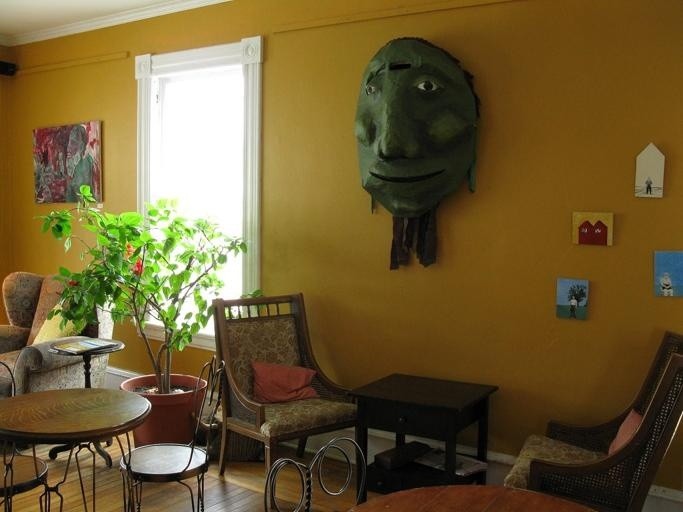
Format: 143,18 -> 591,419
503,331 -> 683,512
120,355 -> 226,512
0,272 -> 115,397
208,294 -> 359,512
0,361 -> 51,512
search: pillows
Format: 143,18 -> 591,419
25,276 -> 88,345
251,362 -> 321,404
608,409 -> 643,457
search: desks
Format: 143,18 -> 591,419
343,484 -> 600,512
0,387 -> 153,512
349,372 -> 500,505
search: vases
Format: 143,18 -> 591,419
120,372 -> 209,449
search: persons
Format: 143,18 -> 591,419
62,126 -> 94,200
353,35 -> 484,222
569,295 -> 579,318
644,177 -> 653,194
660,272 -> 674,296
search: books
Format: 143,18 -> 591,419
47,337 -> 119,357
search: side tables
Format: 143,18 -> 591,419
49,338 -> 126,467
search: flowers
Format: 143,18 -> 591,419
33,185 -> 268,393
